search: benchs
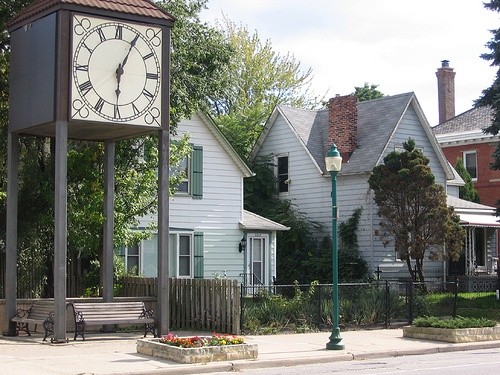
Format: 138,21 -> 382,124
10,301 -> 54,340
67,302 -> 156,340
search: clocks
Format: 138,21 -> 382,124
68,15 -> 163,128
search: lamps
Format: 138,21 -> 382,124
239,237 -> 248,254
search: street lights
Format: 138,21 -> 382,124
324,142 -> 344,350
